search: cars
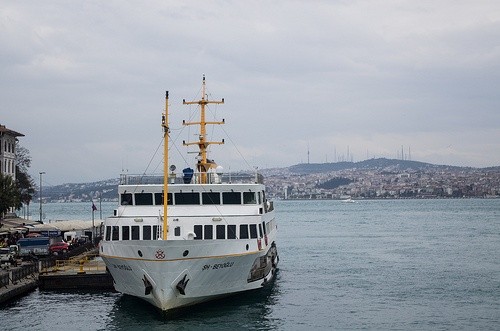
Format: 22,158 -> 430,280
0,240 -> 74,266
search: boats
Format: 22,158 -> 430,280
341,198 -> 355,203
99,74 -> 280,313
